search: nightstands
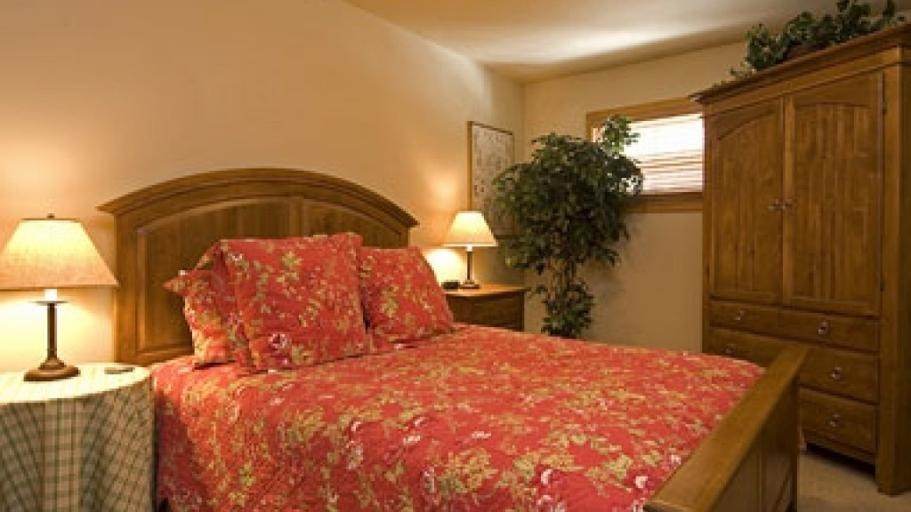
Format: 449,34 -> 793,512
442,282 -> 531,331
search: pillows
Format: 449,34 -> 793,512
166,234 -> 457,373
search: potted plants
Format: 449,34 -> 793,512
738,0 -> 905,68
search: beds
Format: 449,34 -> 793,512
97,168 -> 810,512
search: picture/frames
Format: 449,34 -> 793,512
467,120 -> 515,239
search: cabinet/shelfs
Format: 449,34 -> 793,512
690,22 -> 911,497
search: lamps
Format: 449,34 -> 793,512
442,211 -> 499,289
0,214 -> 120,382
